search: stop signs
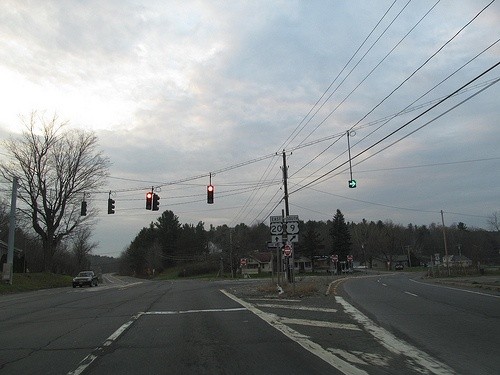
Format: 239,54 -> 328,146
332,256 -> 338,261
347,256 -> 353,261
284,245 -> 292,256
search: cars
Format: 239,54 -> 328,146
72,271 -> 98,287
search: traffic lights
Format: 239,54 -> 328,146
154,194 -> 160,211
207,185 -> 214,203
81,201 -> 87,216
108,198 -> 115,214
146,193 -> 152,210
